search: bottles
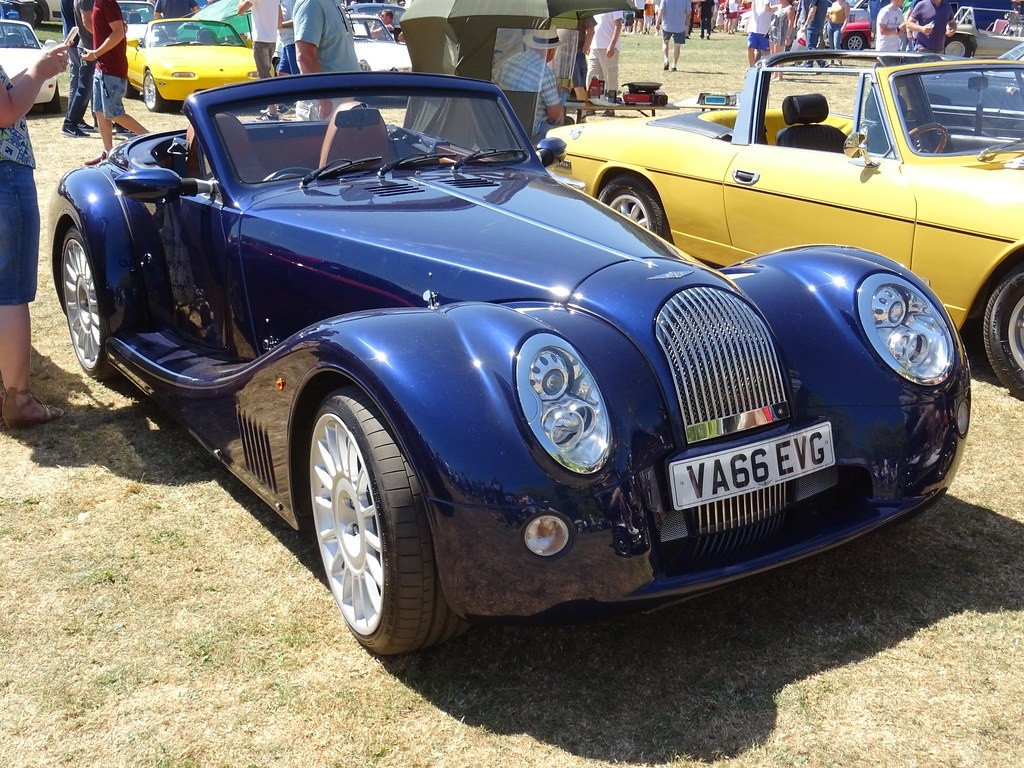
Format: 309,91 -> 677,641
599,80 -> 605,95
608,88 -> 617,103
588,76 -> 601,99
925,20 -> 936,35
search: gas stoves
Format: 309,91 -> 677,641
622,90 -> 668,106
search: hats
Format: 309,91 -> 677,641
522,24 -> 568,49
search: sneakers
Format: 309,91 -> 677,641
84,151 -> 107,167
256,112 -> 279,121
61,120 -> 90,137
74,120 -> 95,131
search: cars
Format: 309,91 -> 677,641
945,6 -> 1024,58
0,17 -> 63,115
840,0 -> 872,51
116,1 -> 165,43
344,3 -> 413,72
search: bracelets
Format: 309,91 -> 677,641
94,52 -> 98,59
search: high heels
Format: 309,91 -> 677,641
3,387 -> 64,429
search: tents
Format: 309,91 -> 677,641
399,0 -> 640,158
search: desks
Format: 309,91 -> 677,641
565,96 -> 738,126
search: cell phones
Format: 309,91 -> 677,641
63,27 -> 79,46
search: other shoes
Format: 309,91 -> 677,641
770,75 -> 783,82
600,109 -> 615,117
817,60 -> 829,74
711,28 -> 736,35
700,34 -> 710,39
277,104 -> 290,116
115,127 -> 137,137
671,68 -> 678,72
623,29 -> 650,36
663,61 -> 670,71
575,108 -> 595,116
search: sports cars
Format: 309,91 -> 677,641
121,18 -> 276,114
51,71 -> 972,659
537,47 -> 1024,400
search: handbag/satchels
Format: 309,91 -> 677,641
790,29 -> 807,53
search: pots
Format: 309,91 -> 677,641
621,81 -> 662,93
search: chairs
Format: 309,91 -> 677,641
6,33 -> 24,47
776,94 -> 849,152
318,100 -> 398,173
184,111 -> 268,185
152,28 -> 171,46
865,91 -> 914,155
130,12 -> 142,23
196,27 -> 220,46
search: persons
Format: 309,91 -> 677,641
60,0 -> 148,164
873,0 -> 957,66
370,9 -> 398,41
686,0 -> 750,39
586,10 -> 623,116
237,0 -> 300,121
493,24 -> 575,145
655,0 -> 692,72
553,15 -> 597,124
0,41 -> 75,425
154,0 -> 199,36
623,0 -> 661,36
293,0 -> 359,120
747,0 -> 850,82
